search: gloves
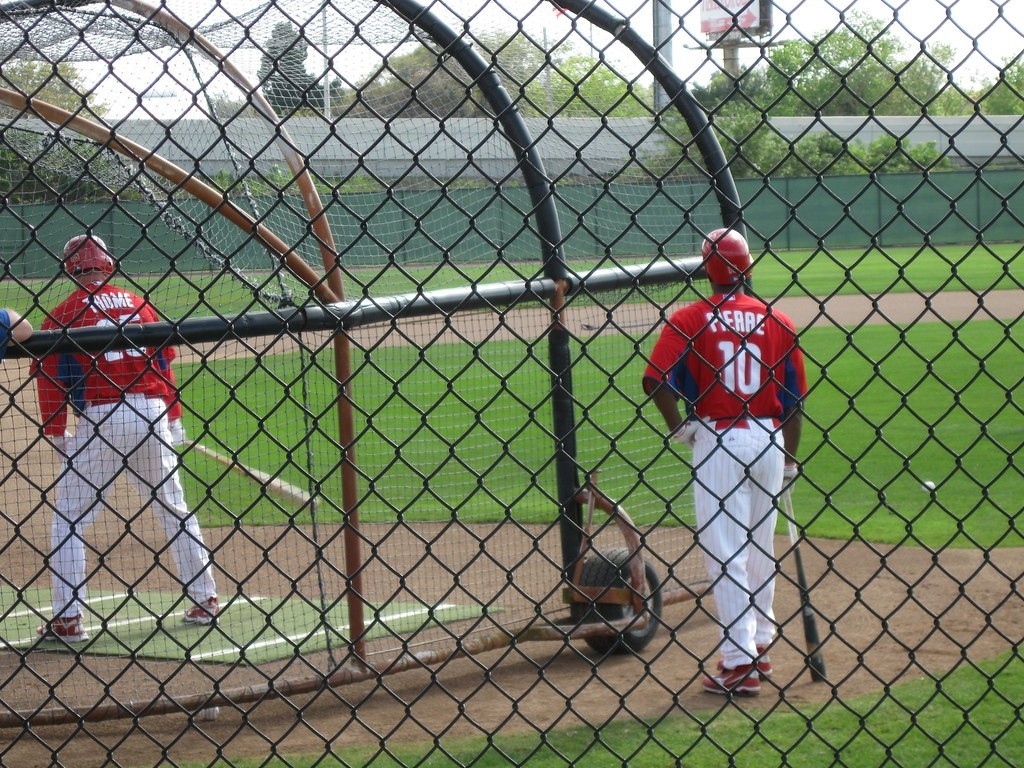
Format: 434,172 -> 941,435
167,417 -> 186,446
782,463 -> 797,494
672,416 -> 711,449
54,430 -> 73,463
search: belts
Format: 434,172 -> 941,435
90,394 -> 163,408
714,417 -> 781,431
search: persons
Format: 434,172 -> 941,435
0,308 -> 33,365
641,228 -> 808,696
29,237 -> 219,640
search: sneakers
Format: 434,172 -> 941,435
702,662 -> 761,693
716,645 -> 774,674
183,595 -> 219,623
37,614 -> 89,642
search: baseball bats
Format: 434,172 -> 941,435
184,438 -> 320,511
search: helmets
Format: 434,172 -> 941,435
63,234 -> 113,276
702,228 -> 754,284
